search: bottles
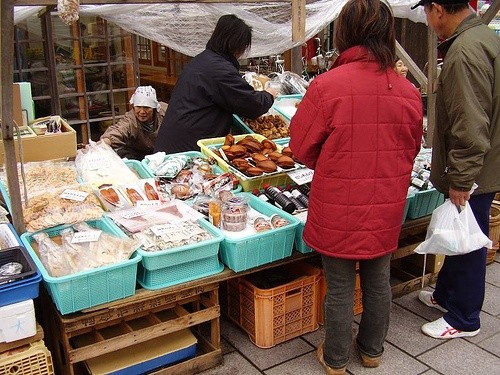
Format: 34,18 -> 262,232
295,184 -> 310,198
286,185 -> 308,208
410,178 -> 428,190
277,186 -> 305,210
263,183 -> 296,214
252,188 -> 275,206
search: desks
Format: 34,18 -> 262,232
38,210 -> 444,375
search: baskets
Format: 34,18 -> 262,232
0,93 -> 445,349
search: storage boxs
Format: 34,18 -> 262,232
0,94 -> 444,375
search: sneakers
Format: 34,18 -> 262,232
418,291 -> 449,313
316,341 -> 347,375
353,334 -> 381,367
421,315 -> 480,339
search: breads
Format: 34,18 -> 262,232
216,134 -> 295,176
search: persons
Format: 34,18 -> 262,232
287,1 -> 424,375
97,85 -> 171,162
24,23 -> 89,112
392,55 -> 411,80
153,14 -> 281,155
418,0 -> 500,341
